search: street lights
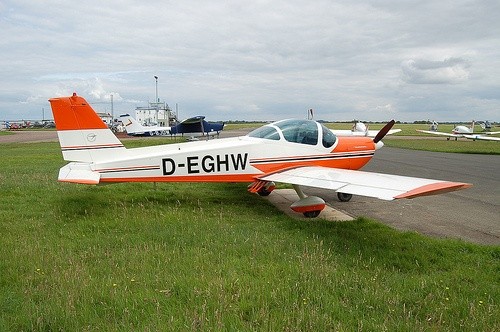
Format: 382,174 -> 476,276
154,76 -> 159,127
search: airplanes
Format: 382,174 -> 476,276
47,92 -> 473,227
480,120 -> 492,130
318,119 -> 401,141
414,117 -> 500,143
2,116 -> 57,129
429,119 -> 440,132
121,112 -> 225,140
